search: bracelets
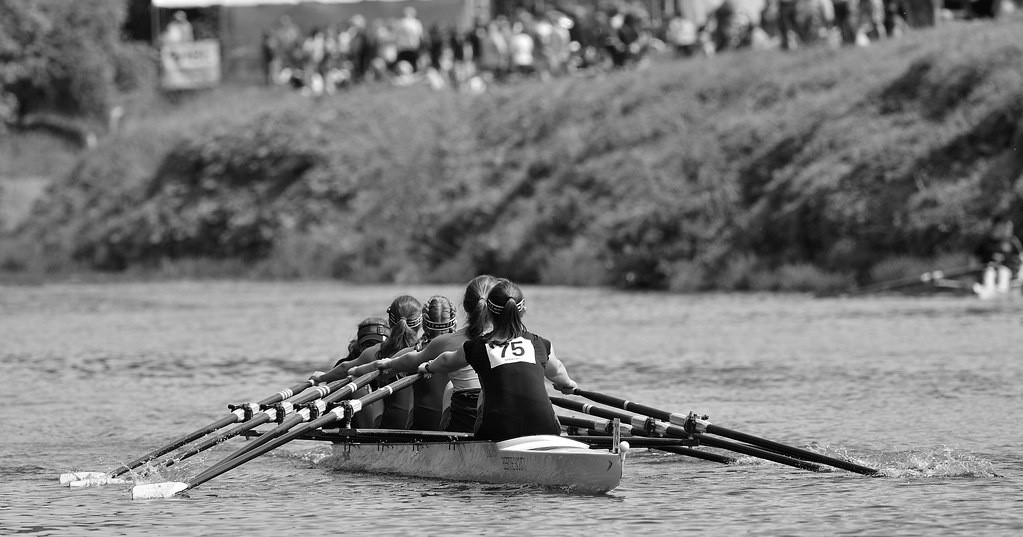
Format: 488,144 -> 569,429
424,364 -> 434,374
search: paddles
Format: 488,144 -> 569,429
60,369 -> 436,500
548,381 -> 882,476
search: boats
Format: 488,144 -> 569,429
331,418 -> 630,494
927,278 -> 1022,303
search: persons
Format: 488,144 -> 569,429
309,275 -> 577,444
971,219 -> 1023,300
